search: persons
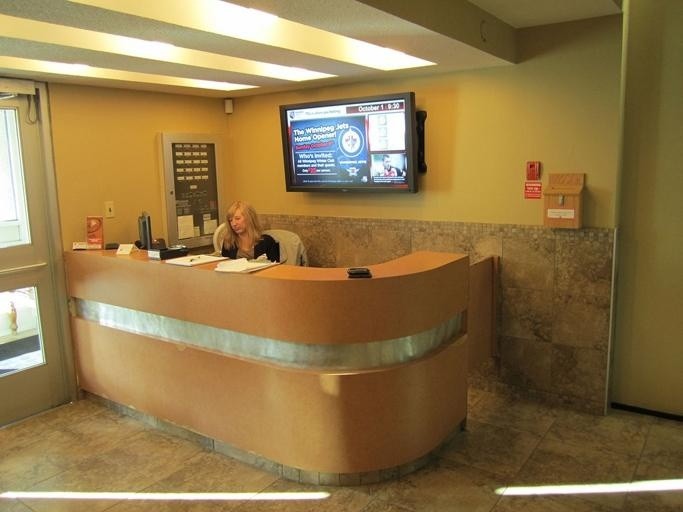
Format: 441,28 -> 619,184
219,201 -> 278,263
375,154 -> 402,177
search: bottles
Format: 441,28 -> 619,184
138,216 -> 151,249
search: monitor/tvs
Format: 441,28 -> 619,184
139,211 -> 152,249
279,92 -> 418,193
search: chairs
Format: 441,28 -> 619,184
263,229 -> 307,265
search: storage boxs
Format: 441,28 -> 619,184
544,172 -> 584,229
